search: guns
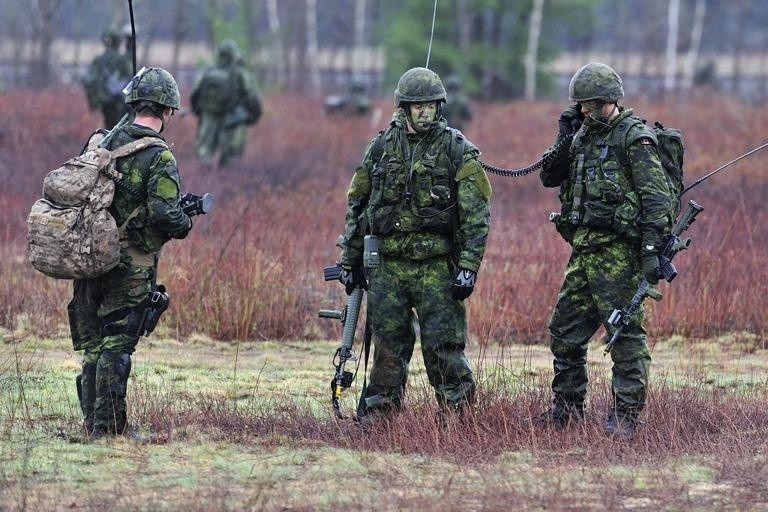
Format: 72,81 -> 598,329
179,192 -> 213,216
317,213 -> 375,398
145,291 -> 170,335
604,199 -> 704,355
549,212 -> 572,247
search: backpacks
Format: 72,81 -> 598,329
83,61 -> 119,111
654,121 -> 684,222
199,69 -> 233,115
26,134 -> 152,280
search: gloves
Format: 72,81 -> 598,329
339,265 -> 368,294
641,253 -> 663,284
559,105 -> 582,134
451,270 -> 475,300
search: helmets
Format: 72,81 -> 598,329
123,67 -> 181,110
394,66 -> 447,108
220,40 -> 242,62
568,64 -> 624,103
104,31 -> 120,47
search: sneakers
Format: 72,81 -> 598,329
534,404 -> 586,429
607,415 -> 635,441
84,419 -> 149,445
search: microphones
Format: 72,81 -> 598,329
423,113 -> 444,127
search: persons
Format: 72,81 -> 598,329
81,28 -> 130,131
539,63 -> 672,442
440,76 -> 472,134
67,66 -> 192,442
188,38 -> 263,172
340,67 -> 491,426
